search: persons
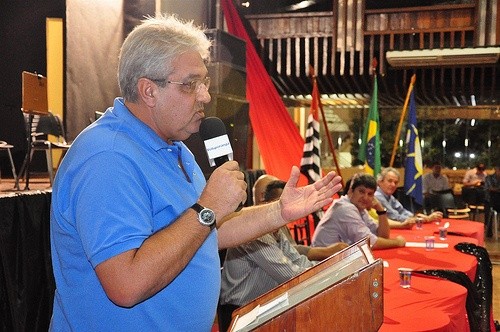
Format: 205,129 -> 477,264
217,159 -> 500,332
48,14 -> 342,332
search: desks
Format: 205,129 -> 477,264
372,219 -> 496,332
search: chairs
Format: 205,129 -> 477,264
14,107 -> 72,188
0,141 -> 21,191
432,185 -> 499,241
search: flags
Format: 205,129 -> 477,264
358,75 -> 382,178
300,80 -> 322,184
404,90 -> 424,205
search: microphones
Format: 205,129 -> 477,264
199,117 -> 243,212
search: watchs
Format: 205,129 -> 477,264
376,207 -> 387,215
191,203 -> 217,231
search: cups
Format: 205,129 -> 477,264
425,236 -> 435,251
440,227 -> 448,240
398,268 -> 413,288
416,218 -> 424,229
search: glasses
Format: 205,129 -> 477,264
152,77 -> 211,93
